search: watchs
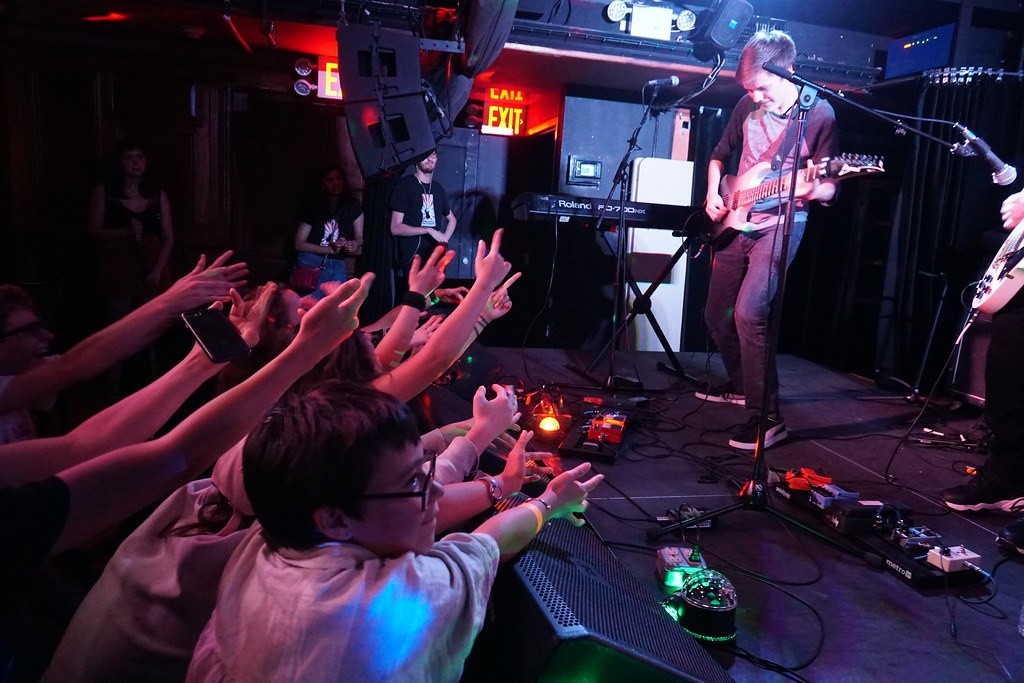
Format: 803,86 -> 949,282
478,475 -> 504,506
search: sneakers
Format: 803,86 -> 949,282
729,413 -> 787,449
995,517 -> 1024,555
939,466 -> 1024,512
695,382 -> 746,404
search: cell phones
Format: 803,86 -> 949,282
182,308 -> 253,364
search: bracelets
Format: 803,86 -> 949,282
405,290 -> 427,311
526,497 -> 551,512
523,504 -> 542,533
474,315 -> 489,336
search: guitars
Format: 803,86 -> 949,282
969,218 -> 1024,317
694,151 -> 885,250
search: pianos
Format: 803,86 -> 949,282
510,187 -> 715,387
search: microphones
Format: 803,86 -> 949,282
645,76 -> 680,86
951,121 -> 1017,185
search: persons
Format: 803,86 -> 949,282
941,186 -> 1024,514
0,226 -> 520,683
185,383 -> 603,683
390,148 -> 456,309
88,144 -> 173,326
704,30 -> 837,449
294,167 -> 365,309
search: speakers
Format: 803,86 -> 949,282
439,472 -> 736,683
948,307 -> 992,406
406,379 -> 556,496
703,0 -> 754,51
335,19 -> 437,186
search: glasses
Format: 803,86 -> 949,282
428,153 -> 439,160
349,449 -> 437,510
0,320 -> 45,342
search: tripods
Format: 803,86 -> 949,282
643,61 -> 979,569
